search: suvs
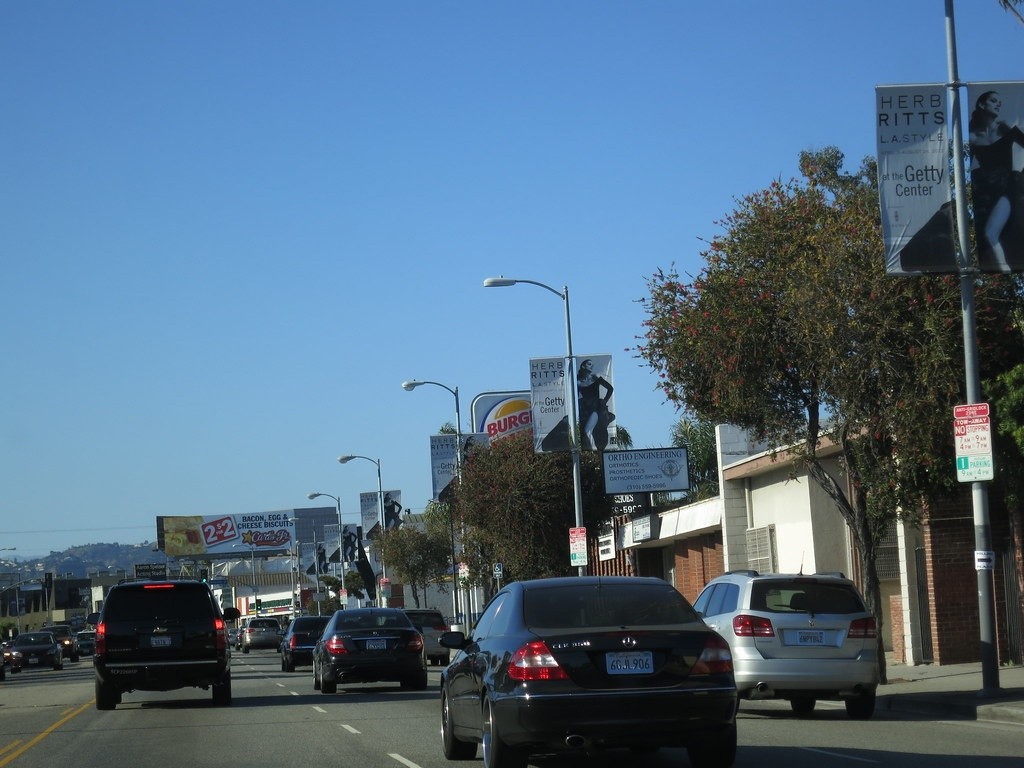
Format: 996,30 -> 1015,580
85,576 -> 241,711
691,569 -> 889,719
399,608 -> 451,667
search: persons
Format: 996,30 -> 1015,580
461,435 -> 479,465
343,525 -> 358,562
384,492 -> 402,530
969,91 -> 1024,270
317,544 -> 328,574
577,359 -> 614,450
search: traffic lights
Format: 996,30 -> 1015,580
256,599 -> 261,611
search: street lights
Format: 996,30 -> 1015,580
401,380 -> 473,640
306,492 -> 347,611
232,544 -> 258,617
483,277 -> 589,577
295,540 -> 302,616
336,455 -> 388,608
287,516 -> 322,616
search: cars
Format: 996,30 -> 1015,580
0,612 -> 100,683
228,617 -> 283,654
307,607 -> 428,694
437,576 -> 738,768
276,615 -> 333,673
